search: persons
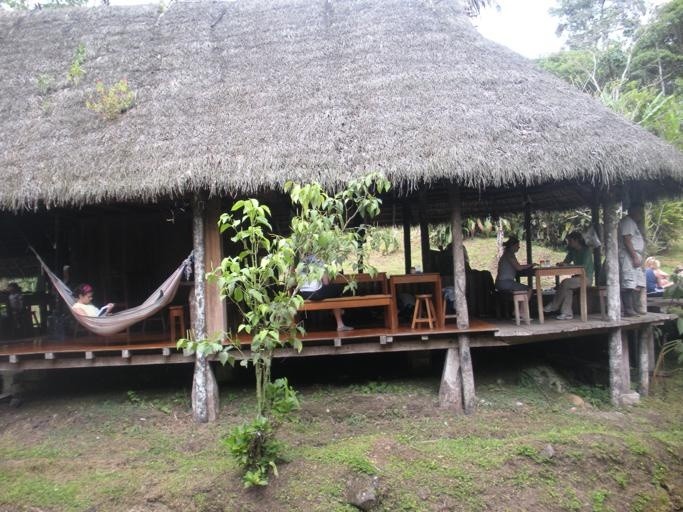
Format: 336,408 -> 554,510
644,256 -> 683,294
617,202 -> 648,317
5,282 -> 24,329
70,282 -> 163,337
293,244 -> 354,332
493,235 -> 539,321
542,230 -> 594,320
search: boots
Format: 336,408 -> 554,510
621,285 -> 647,317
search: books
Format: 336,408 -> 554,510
96,302 -> 113,317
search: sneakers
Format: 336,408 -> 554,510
544,302 -> 559,313
556,313 -> 573,320
337,325 -> 354,332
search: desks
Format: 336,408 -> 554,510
335,271 -> 443,330
518,265 -> 588,324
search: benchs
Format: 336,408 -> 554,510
588,285 -> 607,320
496,290 -> 530,326
297,294 -> 395,331
